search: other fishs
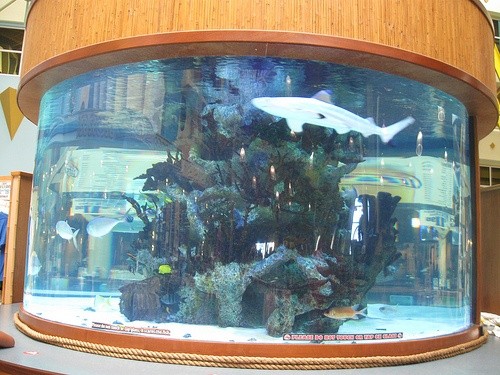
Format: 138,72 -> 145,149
93,294 -> 112,306
27,251 -> 42,275
56,220 -> 80,248
159,265 -> 173,275
65,161 -> 80,178
323,303 -> 371,320
86,208 -> 131,239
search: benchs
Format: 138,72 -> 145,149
365,287 -> 437,305
